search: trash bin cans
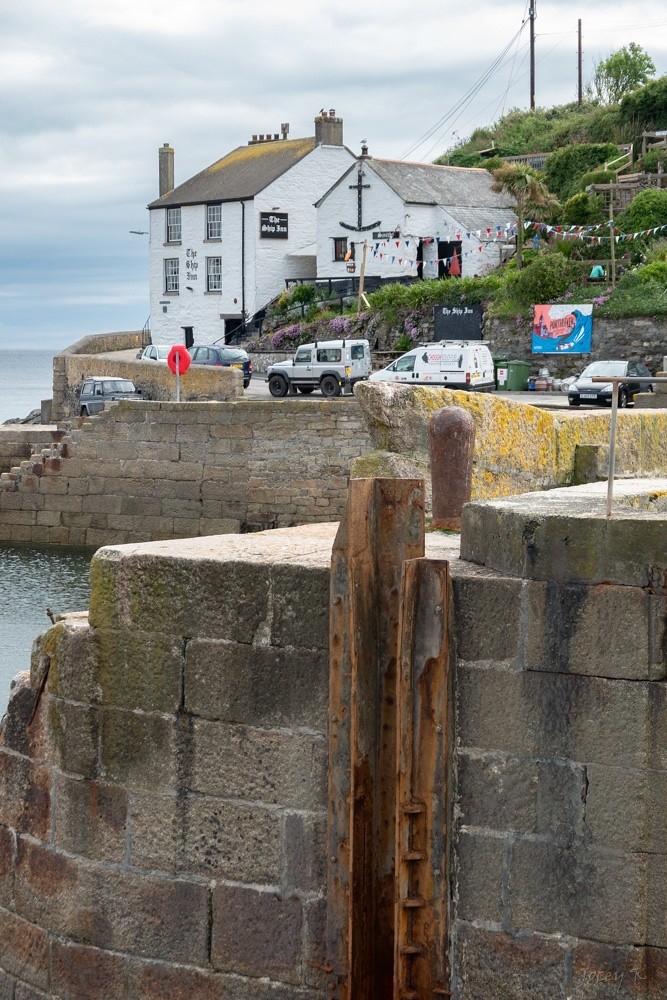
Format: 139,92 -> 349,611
492,356 -> 531,391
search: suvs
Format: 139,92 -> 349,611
141,344 -> 173,367
367,339 -> 497,393
185,344 -> 254,389
77,375 -> 149,418
264,339 -> 372,398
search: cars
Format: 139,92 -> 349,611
568,360 -> 654,409
